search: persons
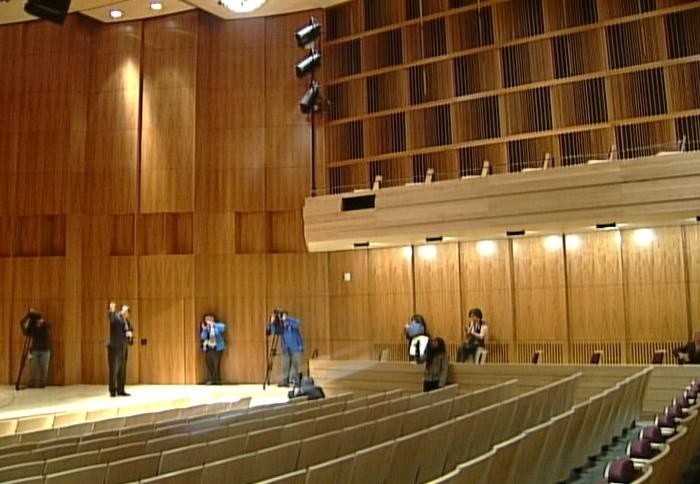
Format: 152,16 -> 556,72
672,332 -> 700,364
288,376 -> 326,401
20,315 -> 52,388
404,314 -> 426,360
456,308 -> 489,364
416,336 -> 449,392
199,314 -> 227,384
107,301 -> 135,396
265,310 -> 304,388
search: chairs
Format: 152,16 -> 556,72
264,413 -> 294,427
468,402 -> 501,459
99,441 -> 146,464
402,404 -> 432,435
390,396 -> 411,415
121,422 -> 155,435
638,424 -> 688,484
0,435 -> 20,447
104,452 -> 161,484
205,433 -> 248,464
511,421 -> 554,484
0,452 -> 31,467
603,458 -> 653,484
683,379 -> 700,398
60,423 -> 93,437
384,429 -> 429,484
316,412 -> 344,434
672,396 -> 700,446
368,392 -> 386,404
256,468 -> 307,484
443,410 -> 483,474
444,383 -> 458,398
410,391 -> 429,409
371,411 -> 406,445
294,406 -> 321,421
602,381 -> 629,445
337,420 -> 377,457
532,350 -> 541,364
452,378 -> 520,418
429,388 -> 445,402
534,410 -> 574,484
0,441 -> 38,455
626,439 -> 671,483
348,439 -> 396,484
624,371 -> 644,428
346,397 -> 368,410
190,424 -> 229,444
158,442 -> 208,475
257,440 -> 302,481
296,430 -> 342,470
38,435 -> 81,448
119,429 -> 156,445
201,452 -> 257,484
590,351 -> 602,364
426,469 -> 463,484
305,453 -> 356,484
655,409 -> 698,473
487,432 -> 526,484
124,481 -> 140,484
634,367 -> 652,420
491,371 -> 583,445
572,393 -> 606,467
145,433 -> 190,454
229,418 -> 264,435
45,463 -> 108,484
344,406 -> 369,426
156,422 -> 190,438
245,425 -> 283,453
652,349 -> 665,364
0,460 -> 46,482
284,419 -> 316,442
140,465 -> 204,484
21,429 -> 59,442
614,376 -> 637,436
428,398 -> 453,426
31,442 -> 78,461
44,449 -> 99,474
0,475 -> 45,484
368,401 -> 390,420
415,419 -> 457,484
588,386 -> 620,456
386,388 -> 403,400
94,418 -> 123,432
321,400 -> 346,415
124,413 -> 154,427
553,401 -> 592,482
77,436 -> 118,452
81,428 -> 119,441
458,450 -> 496,483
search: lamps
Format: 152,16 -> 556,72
154,391 -> 355,430
25,0 -> 71,24
295,15 -> 322,46
299,79 -> 319,113
296,47 -> 321,74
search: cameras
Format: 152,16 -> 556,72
273,306 -> 288,321
203,321 -> 209,328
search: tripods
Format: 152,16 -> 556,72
16,336 -> 33,390
262,321 -> 300,390
